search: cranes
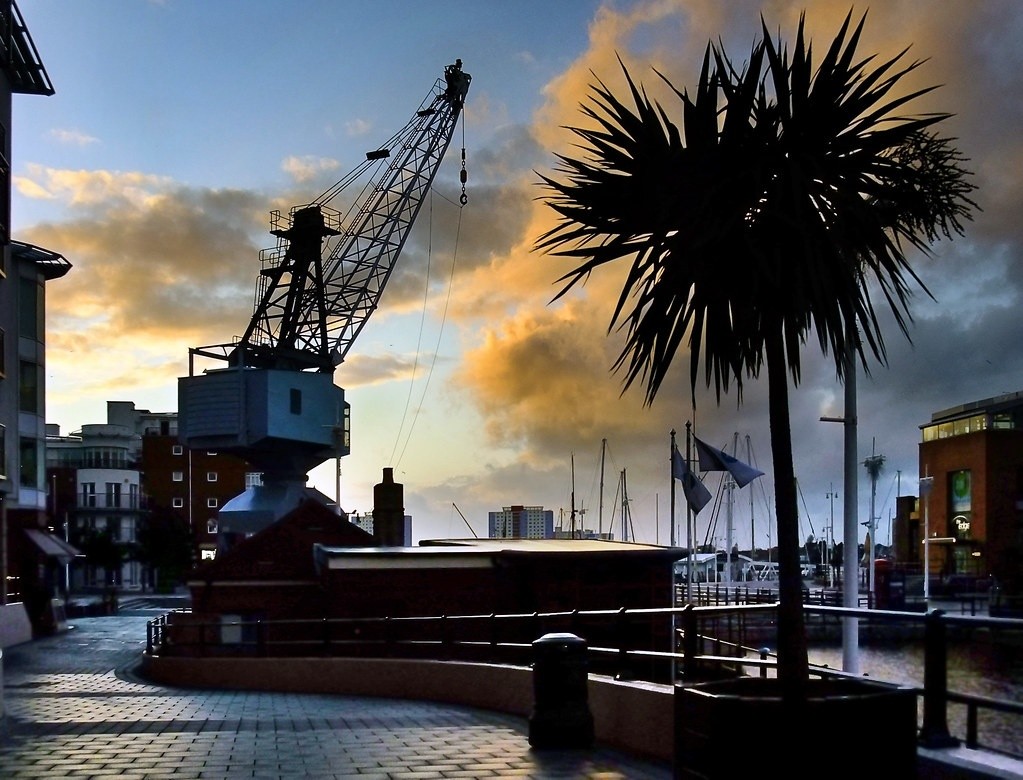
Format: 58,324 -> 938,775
176,59 -> 473,559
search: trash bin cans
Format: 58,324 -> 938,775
529,634 -> 596,748
867,566 -> 906,611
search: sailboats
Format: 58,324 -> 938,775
554,421 -> 901,605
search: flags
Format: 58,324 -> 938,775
695,437 -> 765,488
673,448 -> 712,515
